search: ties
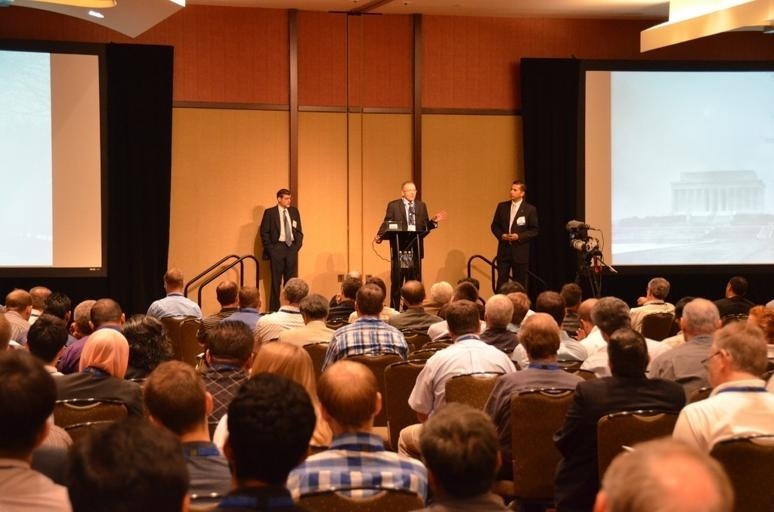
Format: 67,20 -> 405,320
508,201 -> 516,245
408,200 -> 414,225
283,210 -> 292,247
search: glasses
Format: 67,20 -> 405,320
700,349 -> 729,369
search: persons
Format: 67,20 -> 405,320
375,180 -> 448,312
490,179 -> 540,296
1,268 -> 774,512
260,187 -> 304,313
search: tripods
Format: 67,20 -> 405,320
573,262 -> 600,298
